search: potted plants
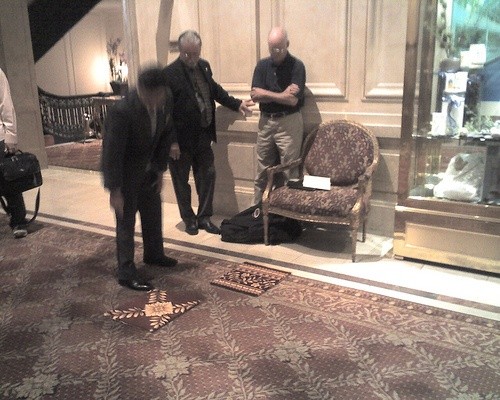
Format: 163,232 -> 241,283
106,34 -> 129,96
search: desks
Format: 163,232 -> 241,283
92,95 -> 124,139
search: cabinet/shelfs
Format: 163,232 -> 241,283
389,1 -> 500,279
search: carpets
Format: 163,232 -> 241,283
0,205 -> 500,400
44,137 -> 109,172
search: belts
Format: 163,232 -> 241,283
261,107 -> 299,118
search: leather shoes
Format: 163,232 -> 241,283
142,255 -> 178,267
119,276 -> 154,291
185,220 -> 199,235
197,217 -> 221,234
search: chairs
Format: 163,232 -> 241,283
261,119 -> 381,263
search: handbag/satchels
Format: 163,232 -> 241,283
0,149 -> 43,198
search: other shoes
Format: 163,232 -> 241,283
11,220 -> 31,238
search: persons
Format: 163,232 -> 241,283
0,68 -> 27,237
250,25 -> 307,211
162,29 -> 256,235
101,59 -> 177,290
